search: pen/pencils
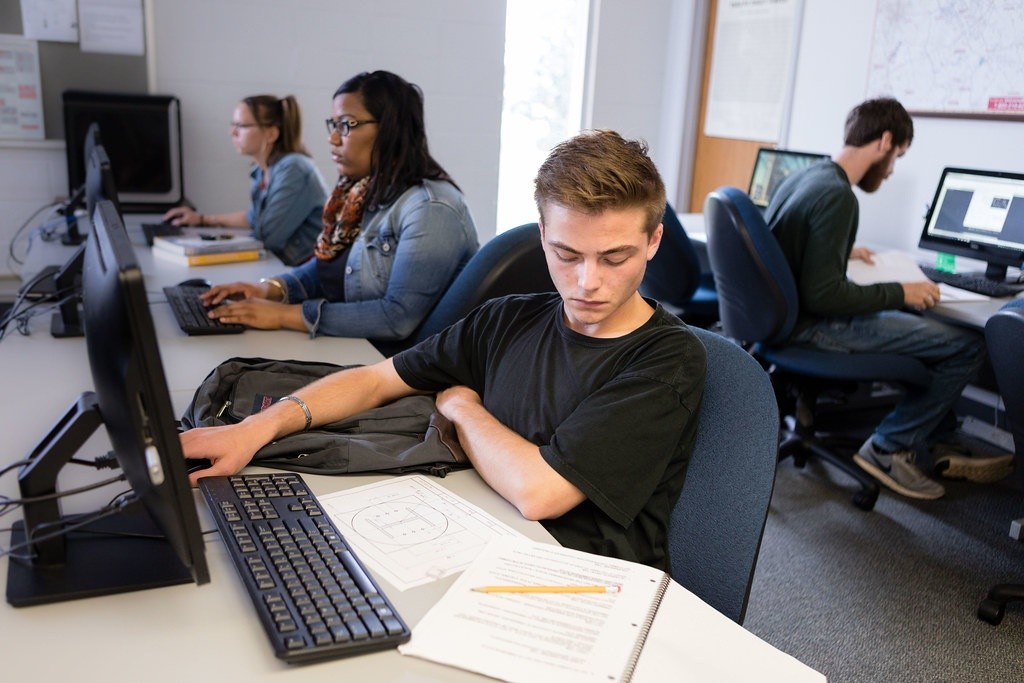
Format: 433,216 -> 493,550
471,586 -> 620,594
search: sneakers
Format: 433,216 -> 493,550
932,442 -> 1017,483
853,435 -> 945,499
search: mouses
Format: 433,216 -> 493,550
164,217 -> 189,227
184,458 -> 212,476
178,279 -> 212,288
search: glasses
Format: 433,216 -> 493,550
326,117 -> 379,136
232,120 -> 279,132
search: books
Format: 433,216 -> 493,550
397,536 -> 828,683
152,236 -> 267,267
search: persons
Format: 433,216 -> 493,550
759,96 -> 980,500
176,129 -> 708,582
162,92 -> 329,268
199,69 -> 480,361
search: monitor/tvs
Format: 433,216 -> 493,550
7,120 -> 212,610
747,148 -> 832,218
917,167 -> 1024,278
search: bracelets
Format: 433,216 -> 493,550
278,395 -> 312,434
259,277 -> 285,299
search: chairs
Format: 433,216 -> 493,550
704,186 -> 933,512
637,200 -> 720,331
389,222 -> 560,359
669,323 -> 778,626
976,308 -> 1024,625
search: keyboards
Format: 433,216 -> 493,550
919,264 -> 1015,298
162,285 -> 246,336
197,473 -> 411,665
141,223 -> 182,246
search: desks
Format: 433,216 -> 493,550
0,198 -> 562,683
675,213 -> 1024,454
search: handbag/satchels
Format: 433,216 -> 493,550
180,356 -> 475,479
